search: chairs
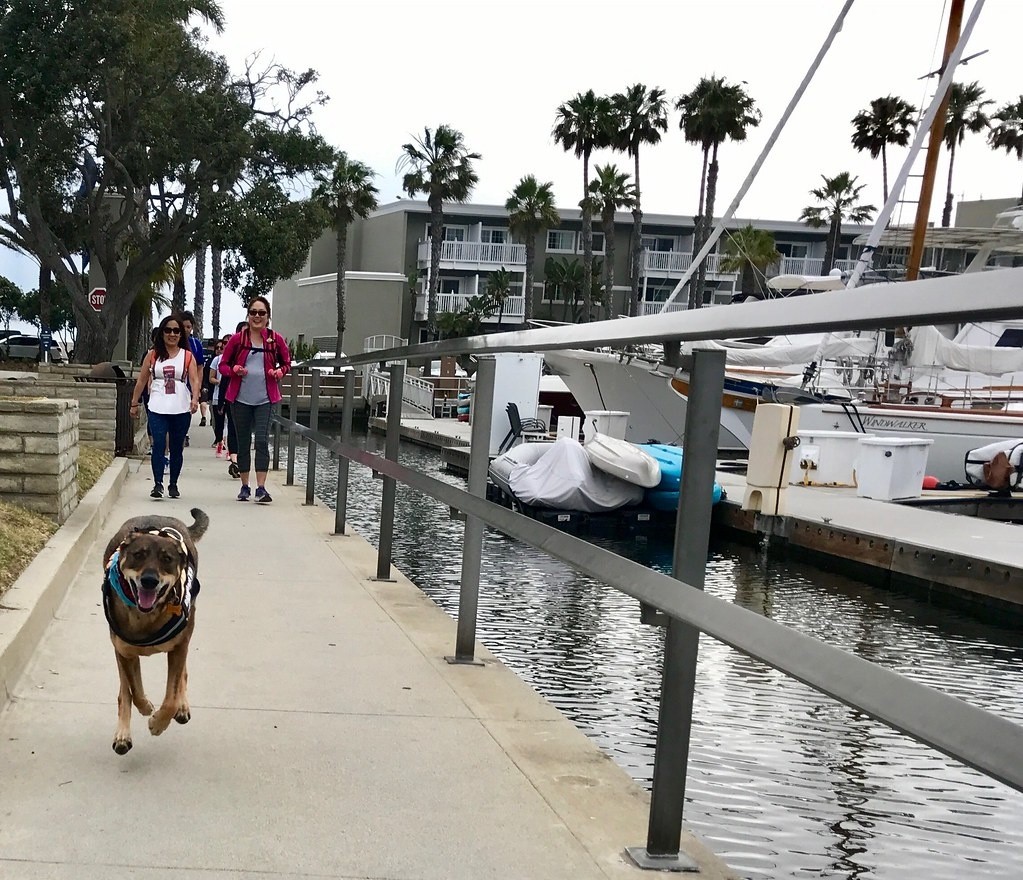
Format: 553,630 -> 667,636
497,402 -> 546,456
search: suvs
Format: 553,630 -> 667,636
0,334 -> 64,364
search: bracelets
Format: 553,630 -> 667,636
131,405 -> 138,407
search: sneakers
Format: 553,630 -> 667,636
167,485 -> 181,498
254,488 -> 272,502
225,451 -> 231,461
236,486 -> 251,501
149,483 -> 165,500
216,441 -> 224,458
229,462 -> 240,479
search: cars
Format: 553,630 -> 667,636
308,351 -> 354,375
0,330 -> 22,341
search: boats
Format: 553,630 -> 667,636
535,1 -> 1022,478
646,480 -> 724,514
630,441 -> 685,489
582,432 -> 663,489
489,436 -> 644,526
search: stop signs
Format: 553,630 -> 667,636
89,287 -> 107,311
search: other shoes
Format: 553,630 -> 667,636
199,418 -> 206,427
184,435 -> 190,447
146,447 -> 153,455
164,457 -> 170,474
212,441 -> 217,448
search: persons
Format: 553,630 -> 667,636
140,311 -> 204,475
219,298 -> 291,503
129,316 -> 199,500
200,322 -> 249,479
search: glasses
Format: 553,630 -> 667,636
215,346 -> 223,350
164,327 -> 180,334
249,310 -> 266,316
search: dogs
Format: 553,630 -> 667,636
101,508 -> 209,755
